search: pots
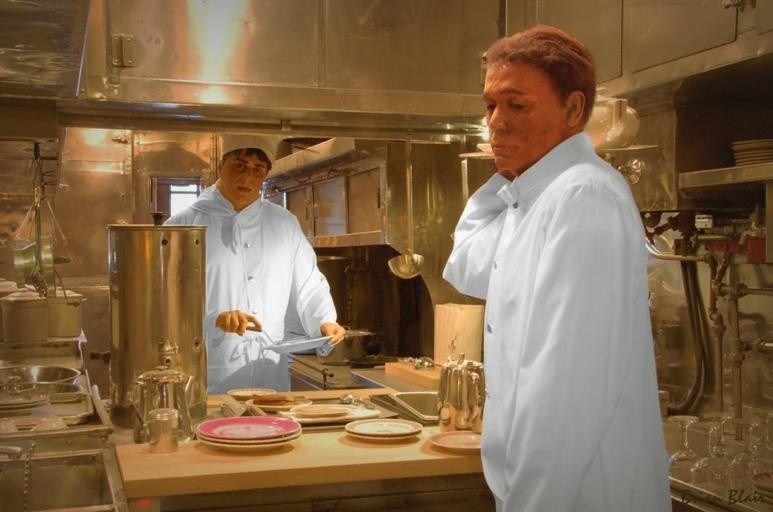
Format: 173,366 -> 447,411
315,324 -> 374,365
0,277 -> 87,342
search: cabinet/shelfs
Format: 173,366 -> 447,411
498,0 -> 772,101
262,136 -> 472,266
63,3 -> 505,155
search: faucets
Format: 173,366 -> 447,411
0,444 -> 26,463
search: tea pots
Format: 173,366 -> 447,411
587,88 -> 642,147
130,367 -> 193,446
436,352 -> 483,431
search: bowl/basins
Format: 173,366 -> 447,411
1,364 -> 80,391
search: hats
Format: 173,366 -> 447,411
219,133 -> 282,168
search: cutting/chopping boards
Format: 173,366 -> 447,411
383,361 -> 445,389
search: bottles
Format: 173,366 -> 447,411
146,406 -> 179,451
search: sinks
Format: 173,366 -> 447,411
1,448 -> 129,512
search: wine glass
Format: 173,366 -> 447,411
668,410 -> 773,506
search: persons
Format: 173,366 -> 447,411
440,24 -> 675,511
160,149 -> 348,396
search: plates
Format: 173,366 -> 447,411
732,137 -> 772,164
228,388 -> 349,417
0,397 -> 50,415
344,418 -> 479,455
194,415 -> 302,451
262,337 -> 331,355
